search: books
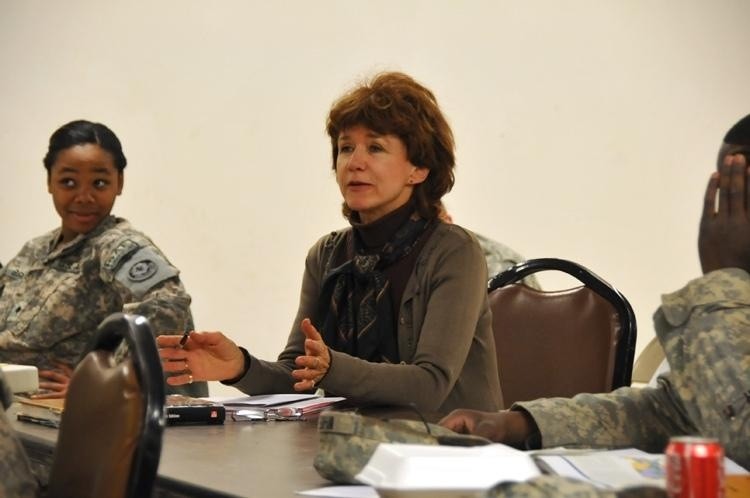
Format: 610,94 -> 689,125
221,390 -> 318,409
223,396 -> 350,423
528,446 -> 748,490
14,392 -> 226,430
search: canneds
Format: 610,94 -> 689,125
664,437 -> 725,498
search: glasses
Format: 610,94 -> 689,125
230,403 -> 308,425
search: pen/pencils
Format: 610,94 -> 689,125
177,334 -> 188,348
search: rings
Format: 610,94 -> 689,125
311,380 -> 316,388
184,359 -> 189,370
189,373 -> 193,384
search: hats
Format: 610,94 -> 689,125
311,406 -> 501,486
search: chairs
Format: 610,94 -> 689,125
488,258 -> 637,409
50,313 -> 166,497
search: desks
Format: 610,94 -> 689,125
6,392 -> 750,497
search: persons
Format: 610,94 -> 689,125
0,119 -> 209,400
155,72 -> 503,427
439,112 -> 748,473
